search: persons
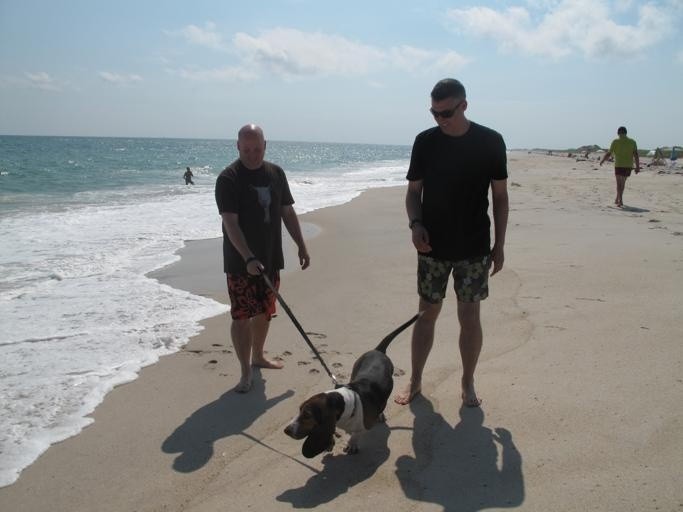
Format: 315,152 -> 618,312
214,124 -> 311,396
390,74 -> 509,408
180,166 -> 197,188
596,126 -> 640,211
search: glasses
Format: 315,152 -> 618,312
429,100 -> 464,119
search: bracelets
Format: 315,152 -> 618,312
406,217 -> 423,230
244,256 -> 258,265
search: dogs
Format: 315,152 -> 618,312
282,309 -> 427,461
